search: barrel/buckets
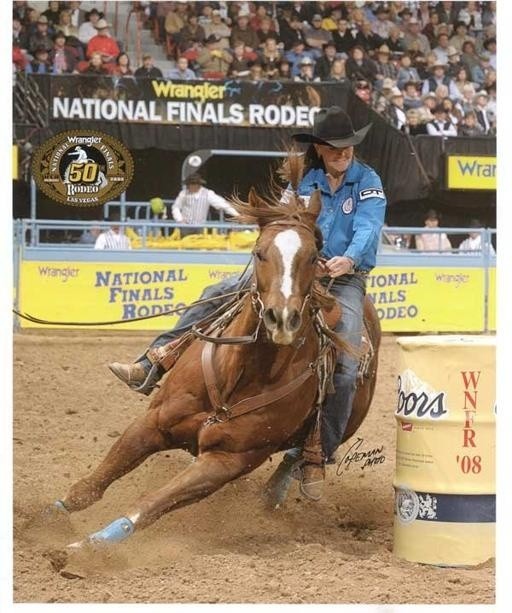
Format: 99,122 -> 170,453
391,335 -> 496,570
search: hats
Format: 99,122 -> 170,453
181,173 -> 208,186
422,208 -> 442,222
104,211 -> 129,222
13,0 -> 497,135
463,218 -> 483,230
293,105 -> 374,148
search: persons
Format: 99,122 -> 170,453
13,1 -> 133,80
349,82 -> 496,139
107,107 -> 387,489
170,174 -> 240,236
78,219 -> 104,243
458,217 -> 495,254
414,207 -> 454,254
94,211 -> 132,249
133,1 -> 496,81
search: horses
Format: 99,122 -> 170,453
43,136 -> 382,573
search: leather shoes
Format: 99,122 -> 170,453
299,447 -> 326,501
108,355 -> 165,396
